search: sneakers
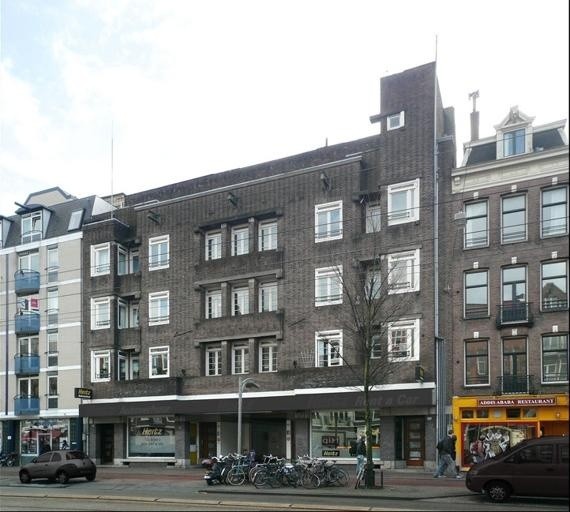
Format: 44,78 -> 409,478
432,472 -> 464,479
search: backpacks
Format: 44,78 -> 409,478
471,441 -> 478,451
436,440 -> 443,453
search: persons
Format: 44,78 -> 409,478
433,430 -> 463,479
355,436 -> 366,479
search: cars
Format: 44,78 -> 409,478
19,450 -> 99,486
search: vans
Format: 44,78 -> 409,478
464,434 -> 570,505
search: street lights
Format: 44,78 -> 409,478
235,376 -> 261,461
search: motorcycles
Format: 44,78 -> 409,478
0,449 -> 18,468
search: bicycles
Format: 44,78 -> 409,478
202,450 -> 369,491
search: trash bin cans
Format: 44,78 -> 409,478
372,460 -> 384,488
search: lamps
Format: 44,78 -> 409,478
454,204 -> 468,228
